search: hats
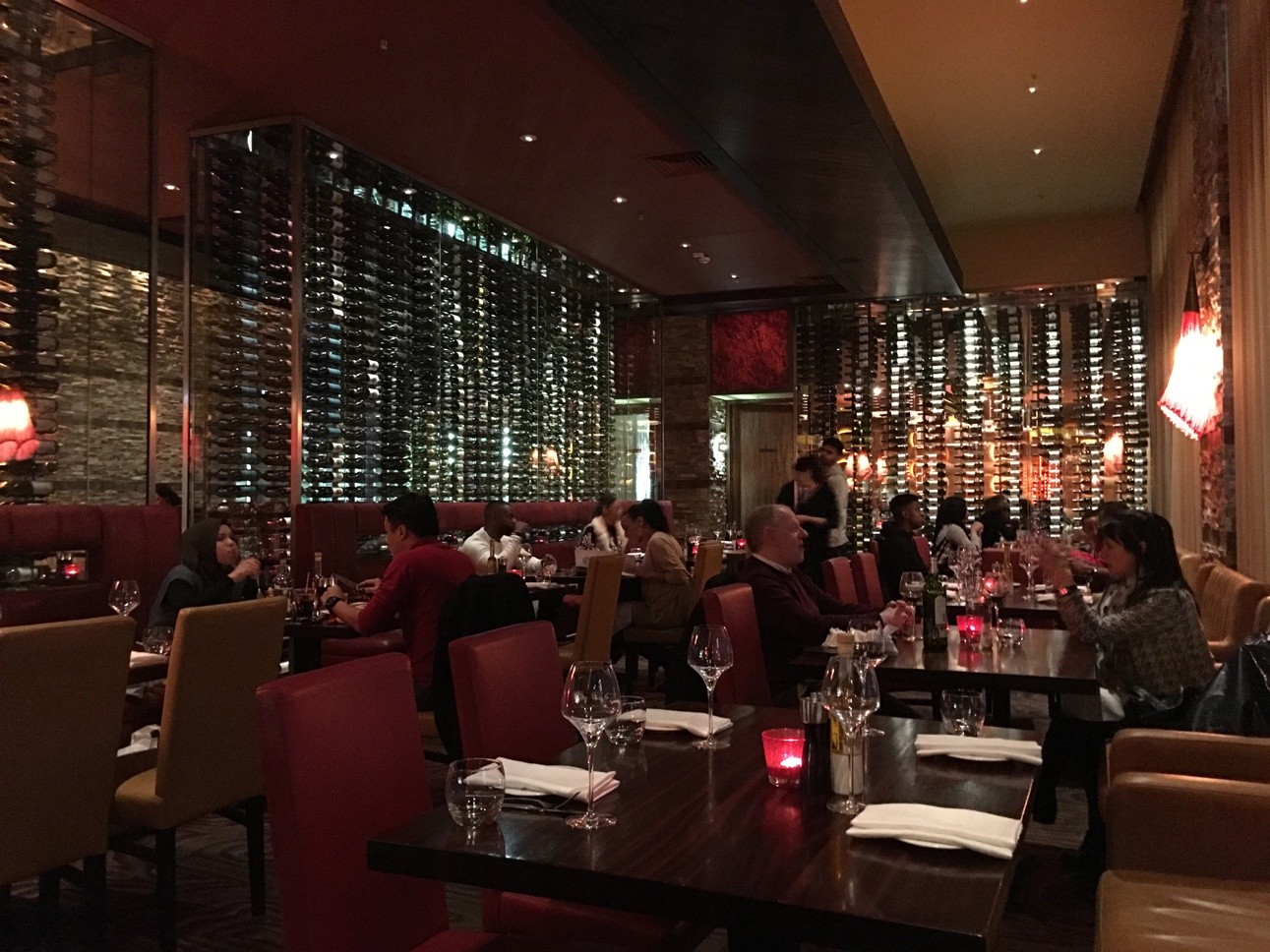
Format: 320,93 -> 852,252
984,495 -> 1007,512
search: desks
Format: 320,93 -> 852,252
0,580 -> 91,593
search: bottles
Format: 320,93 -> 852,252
795,282 -> 1150,557
273,552 -> 325,612
0,1 -> 63,505
194,125 -> 617,602
922,556 -> 992,651
1003,542 -> 1015,594
829,633 -> 863,796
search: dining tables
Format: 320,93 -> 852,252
367,702 -> 1048,952
282,586 -> 401,673
846,621 -> 1102,781
515,569 -> 641,628
918,579 -> 1095,624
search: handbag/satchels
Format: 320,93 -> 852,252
1110,685 -> 1205,735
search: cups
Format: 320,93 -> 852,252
447,758 -> 506,829
941,688 -> 986,739
108,578 -> 175,657
690,521 -> 736,548
801,696 -> 829,742
762,727 -> 805,788
957,614 -> 1026,648
605,696 -> 646,745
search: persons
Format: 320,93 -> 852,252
148,517 -> 261,625
320,492 -> 476,709
778,464 -> 798,511
725,505 -> 925,719
1034,502 -> 1216,823
793,456 -> 841,560
819,437 -> 848,556
980,493 -> 1019,547
609,500 -> 693,664
581,489 -> 629,573
934,496 -> 983,571
879,495 -> 960,608
458,500 -> 563,639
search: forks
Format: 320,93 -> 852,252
504,792 -> 582,808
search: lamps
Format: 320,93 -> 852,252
1158,250 -> 1222,441
0,380 -> 39,461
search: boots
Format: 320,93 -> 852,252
1063,785 -> 1106,883
1031,738 -> 1065,825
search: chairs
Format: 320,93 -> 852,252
0,537 -> 1270,952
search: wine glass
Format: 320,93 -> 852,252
948,544 -> 982,602
560,659 -> 623,829
900,571 -> 924,642
1019,550 -> 1040,600
820,655 -> 883,815
687,624 -> 733,752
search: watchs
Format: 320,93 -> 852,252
325,596 -> 343,615
1054,587 -> 1070,596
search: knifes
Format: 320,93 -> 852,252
502,803 -> 614,817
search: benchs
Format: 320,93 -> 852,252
0,499 -> 178,625
293,500 -> 671,596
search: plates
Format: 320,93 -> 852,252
948,754 -> 1007,761
897,839 -> 963,850
1013,582 -> 1056,602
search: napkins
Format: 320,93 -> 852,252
914,734 -> 1043,766
847,803 -> 1023,859
130,650 -> 167,668
463,757 -> 620,802
1037,594 -> 1093,604
617,708 -> 734,738
